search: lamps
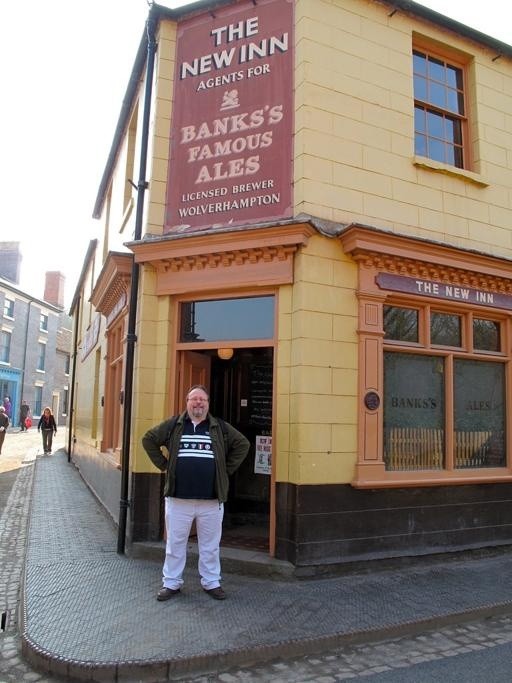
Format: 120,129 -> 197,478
217,349 -> 234,360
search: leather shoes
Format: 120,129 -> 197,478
204,586 -> 227,600
158,587 -> 181,601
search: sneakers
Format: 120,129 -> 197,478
44,452 -> 52,456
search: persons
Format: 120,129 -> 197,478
141,385 -> 252,602
0,406 -> 9,454
38,407 -> 57,455
3,397 -> 11,422
18,400 -> 32,431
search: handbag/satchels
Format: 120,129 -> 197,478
25,417 -> 32,429
